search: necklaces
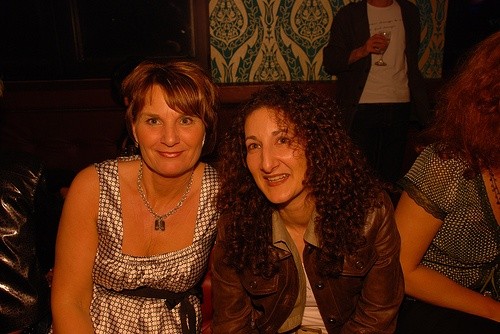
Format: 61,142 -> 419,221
138,159 -> 193,231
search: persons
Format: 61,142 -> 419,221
209,83 -> 405,334
323,0 -> 427,208
51,62 -> 228,334
484,161 -> 500,205
392,32 -> 500,334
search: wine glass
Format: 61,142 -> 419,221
375,31 -> 391,67
